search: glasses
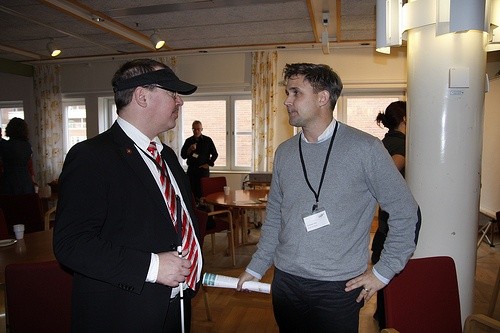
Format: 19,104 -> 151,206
143,85 -> 177,99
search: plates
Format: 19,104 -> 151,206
0,239 -> 17,246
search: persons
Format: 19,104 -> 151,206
181,120 -> 218,203
372,99 -> 407,333
2,116 -> 39,194
51,57 -> 206,333
232,62 -> 422,333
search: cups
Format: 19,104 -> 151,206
224,187 -> 230,195
34,186 -> 38,193
14,225 -> 24,239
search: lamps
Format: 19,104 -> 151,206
46,38 -> 63,56
151,28 -> 165,48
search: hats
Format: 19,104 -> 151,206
113,69 -> 198,95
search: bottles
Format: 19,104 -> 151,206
45,183 -> 51,197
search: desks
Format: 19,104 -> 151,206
0,229 -> 59,333
201,189 -> 269,257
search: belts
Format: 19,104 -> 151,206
168,288 -> 193,307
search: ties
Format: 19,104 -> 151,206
147,142 -> 198,291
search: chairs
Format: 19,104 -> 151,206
375,256 -> 500,333
191,177 -> 236,266
0,183 -> 74,333
